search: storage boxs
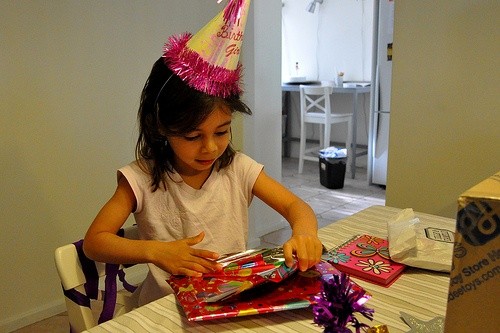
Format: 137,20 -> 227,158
443,170 -> 500,333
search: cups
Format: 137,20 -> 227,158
335,75 -> 343,86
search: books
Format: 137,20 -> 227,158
323,232 -> 408,288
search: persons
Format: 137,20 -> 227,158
82,48 -> 323,310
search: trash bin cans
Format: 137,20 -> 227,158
318,148 -> 348,189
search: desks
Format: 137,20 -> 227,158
78,204 -> 456,333
282,81 -> 371,169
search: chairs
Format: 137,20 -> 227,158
53,222 -> 162,333
298,84 -> 356,179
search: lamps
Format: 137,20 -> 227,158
306,0 -> 324,15
